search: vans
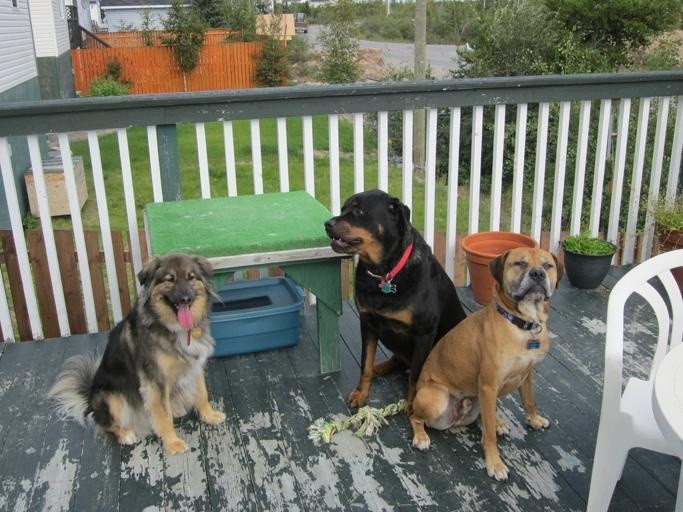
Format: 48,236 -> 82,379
293,12 -> 308,32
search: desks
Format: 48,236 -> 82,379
649,337 -> 682,512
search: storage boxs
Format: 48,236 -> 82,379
24,154 -> 89,219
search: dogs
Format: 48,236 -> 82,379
407,246 -> 564,483
323,187 -> 468,417
45,253 -> 228,457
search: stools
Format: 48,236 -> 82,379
137,188 -> 352,380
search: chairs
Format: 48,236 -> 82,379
582,246 -> 682,510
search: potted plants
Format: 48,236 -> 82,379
619,187 -> 682,288
557,226 -> 615,291
